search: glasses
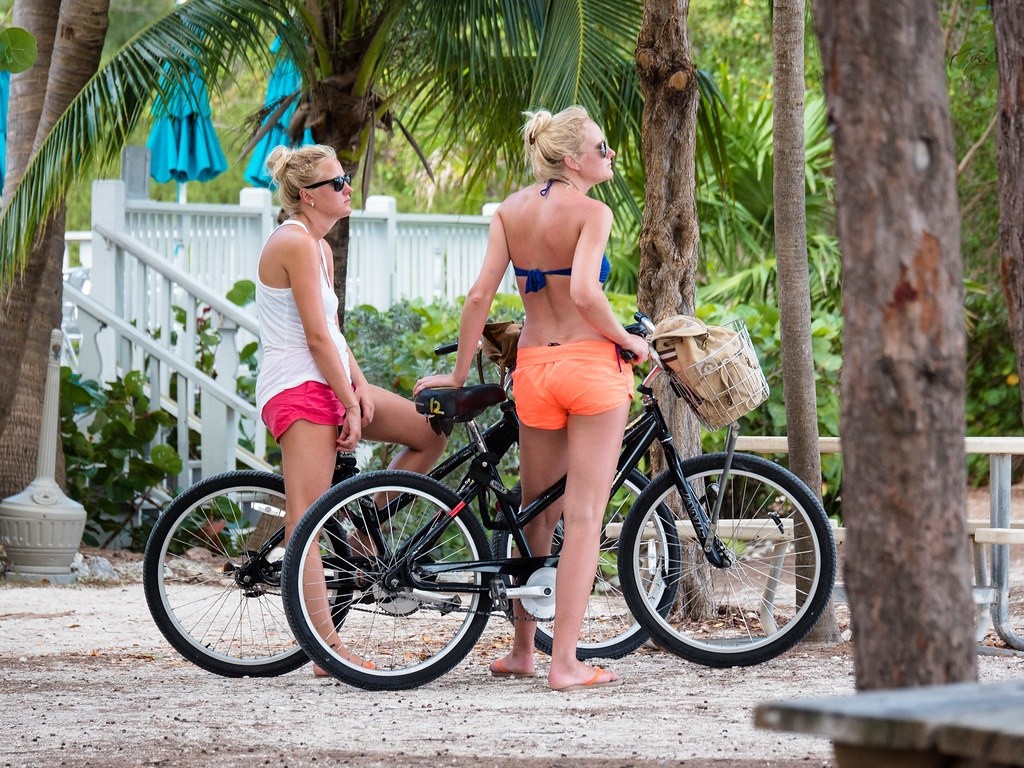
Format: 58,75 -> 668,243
576,141 -> 607,158
296,173 -> 351,200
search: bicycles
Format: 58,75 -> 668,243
278,310 -> 838,692
142,317 -> 684,681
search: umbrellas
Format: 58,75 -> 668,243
148,1 -> 234,205
244,8 -> 320,194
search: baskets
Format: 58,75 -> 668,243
671,318 -> 770,433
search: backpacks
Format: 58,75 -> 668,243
650,314 -> 764,430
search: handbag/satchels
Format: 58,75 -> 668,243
478,321 -> 523,385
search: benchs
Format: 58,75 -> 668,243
752,674 -> 1024,768
604,432 -> 1023,661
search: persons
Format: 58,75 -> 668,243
412,97 -> 652,693
248,142 -> 452,681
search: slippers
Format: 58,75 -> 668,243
493,659 -> 537,677
552,666 -> 623,691
313,663 -> 374,678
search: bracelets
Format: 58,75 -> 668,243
344,404 -> 360,411
